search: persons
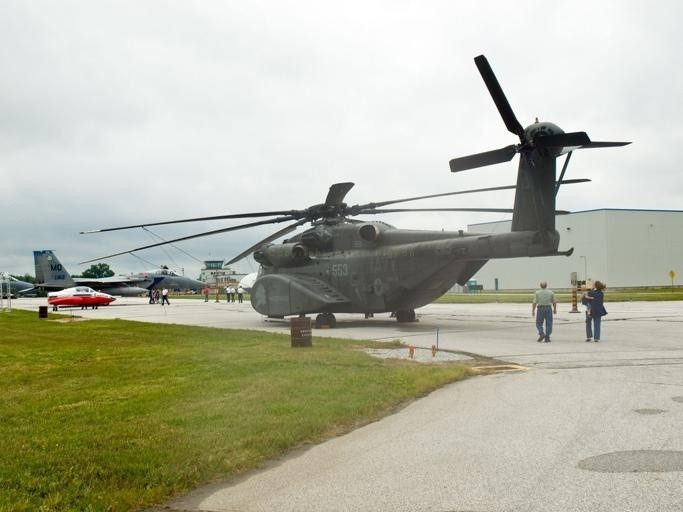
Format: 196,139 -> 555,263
531,280 -> 558,343
161,286 -> 169,305
236,286 -> 244,304
203,286 -> 209,302
581,287 -> 595,320
146,288 -> 161,304
230,285 -> 236,303
224,285 -> 230,302
583,280 -> 608,342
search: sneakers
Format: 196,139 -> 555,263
537,333 -> 551,343
585,338 -> 599,342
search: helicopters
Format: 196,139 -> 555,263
77,54 -> 632,323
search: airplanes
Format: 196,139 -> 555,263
0,250 -> 209,311
